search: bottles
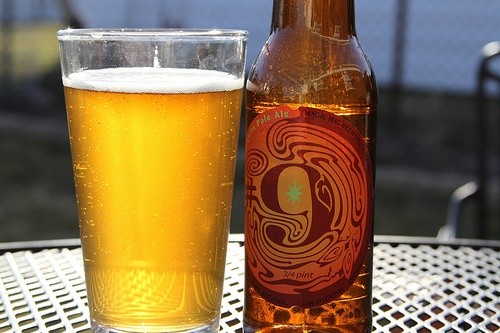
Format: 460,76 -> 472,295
244,0 -> 376,333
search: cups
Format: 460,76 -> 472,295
56,29 -> 249,333
